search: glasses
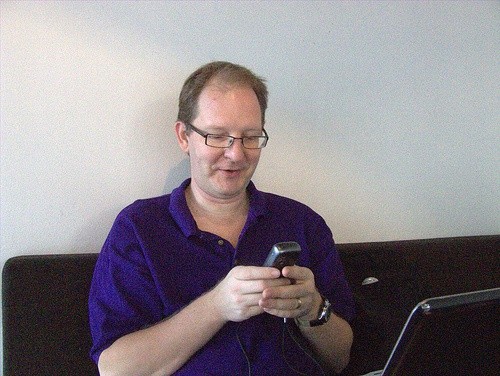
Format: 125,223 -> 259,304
185,121 -> 268,150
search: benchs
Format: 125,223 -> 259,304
1,234 -> 500,376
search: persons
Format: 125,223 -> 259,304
87,60 -> 358,376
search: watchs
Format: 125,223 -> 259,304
297,295 -> 333,327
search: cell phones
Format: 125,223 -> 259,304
263,241 -> 301,277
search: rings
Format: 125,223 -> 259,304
296,299 -> 302,309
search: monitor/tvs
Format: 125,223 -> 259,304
374,287 -> 500,376
